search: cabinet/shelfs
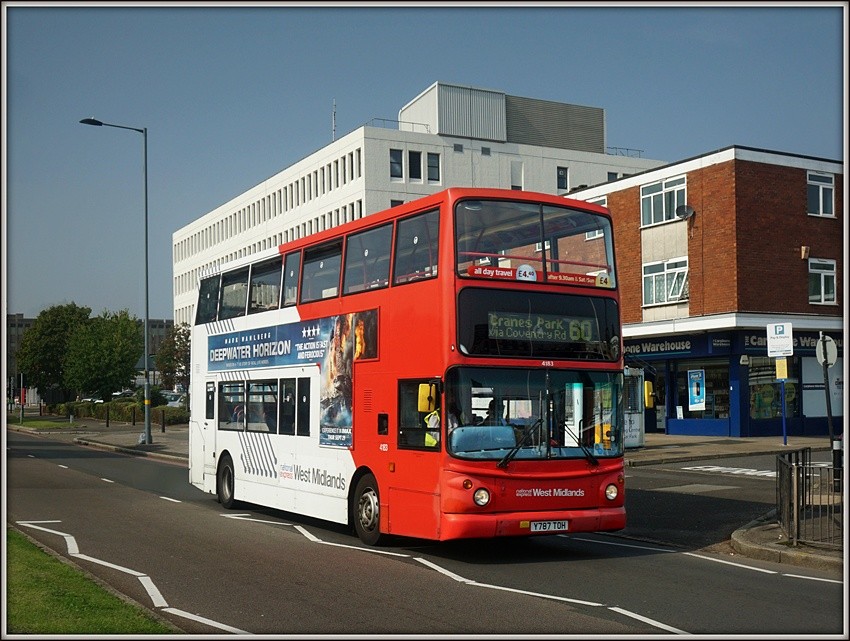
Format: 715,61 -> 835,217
701,393 -> 730,419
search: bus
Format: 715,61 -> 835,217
189,188 -> 655,546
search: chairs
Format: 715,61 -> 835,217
230,405 -> 277,432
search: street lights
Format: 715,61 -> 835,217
77,118 -> 152,444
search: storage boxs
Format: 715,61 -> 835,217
719,413 -> 728,419
715,406 -> 724,411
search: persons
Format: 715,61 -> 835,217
478,400 -> 505,426
424,387 -> 470,447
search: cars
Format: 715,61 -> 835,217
160,390 -> 174,397
113,391 -> 136,400
83,395 -> 96,401
94,393 -> 121,404
165,393 -> 187,407
